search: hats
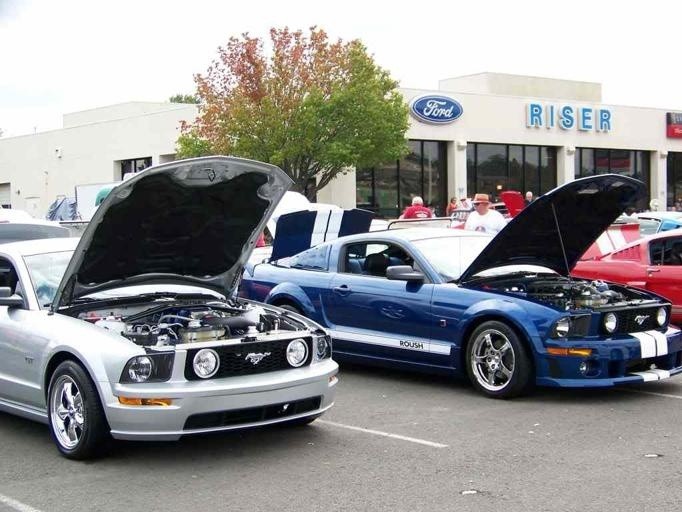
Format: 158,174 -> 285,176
470,193 -> 491,203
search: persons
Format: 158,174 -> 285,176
404,195 -> 433,220
643,199 -> 660,213
463,193 -> 507,234
445,196 -> 459,216
397,206 -> 411,219
671,194 -> 682,212
460,196 -> 466,204
524,191 -> 534,207
422,202 -> 437,218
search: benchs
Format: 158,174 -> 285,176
348,257 -> 405,274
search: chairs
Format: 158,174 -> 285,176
364,253 -> 391,276
8,268 -> 19,294
